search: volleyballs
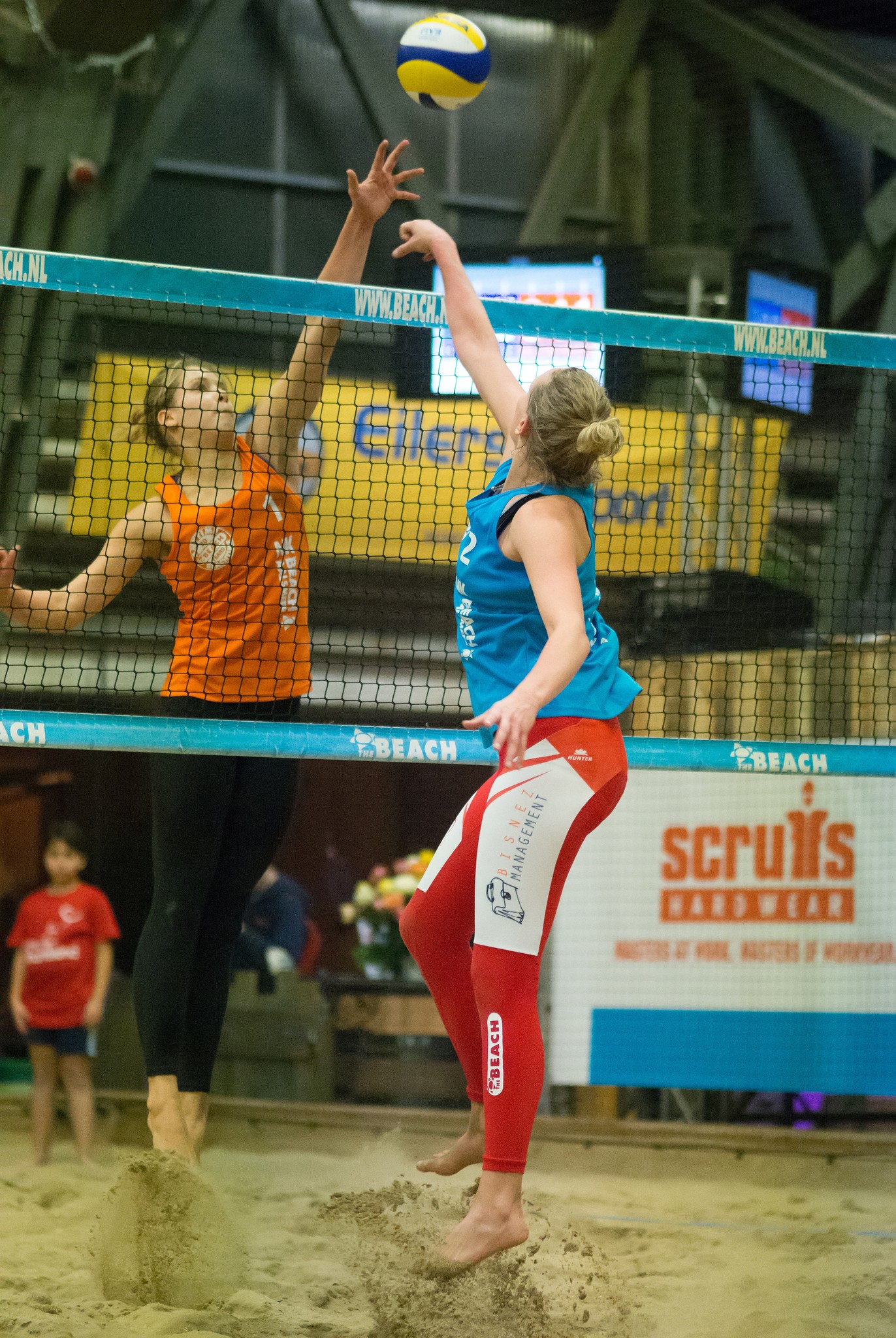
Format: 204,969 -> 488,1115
395,12 -> 492,111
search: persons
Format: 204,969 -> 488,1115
1,141 -> 426,1169
241,862 -> 308,994
390,218 -> 643,1279
7,826 -> 120,1170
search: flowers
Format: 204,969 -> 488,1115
338,848 -> 434,975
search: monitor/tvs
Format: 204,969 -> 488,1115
726,254 -> 835,421
393,245 -> 645,403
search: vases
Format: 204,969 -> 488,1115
356,918 -> 395,980
